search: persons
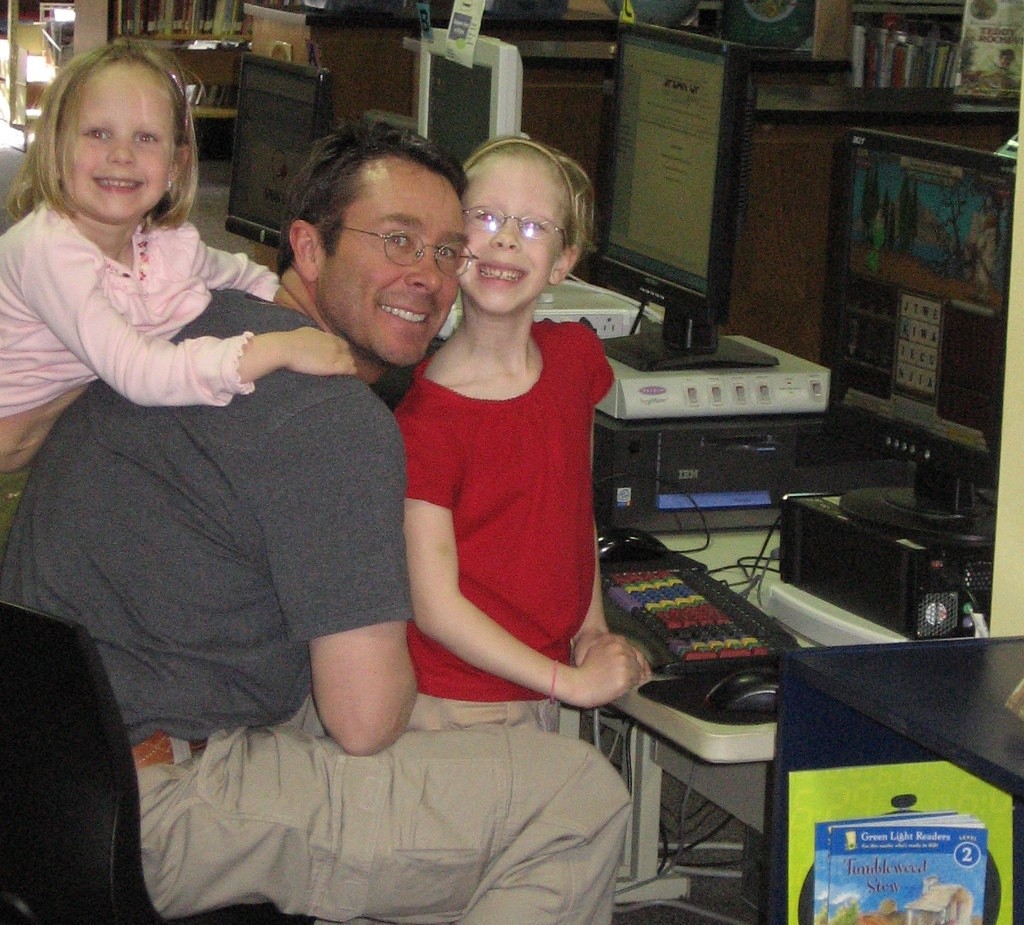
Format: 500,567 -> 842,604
0,40 -> 634,925
394,136 -> 653,731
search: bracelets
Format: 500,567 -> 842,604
549,659 -> 558,704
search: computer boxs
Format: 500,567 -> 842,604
778,493 -> 995,639
585,409 -> 828,534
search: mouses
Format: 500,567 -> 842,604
705,673 -> 784,713
596,525 -> 669,564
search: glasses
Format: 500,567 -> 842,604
317,215 -> 479,277
462,206 -> 568,251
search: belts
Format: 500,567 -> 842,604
129,731 -> 209,768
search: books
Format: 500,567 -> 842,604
814,809 -> 989,925
951,0 -> 1024,100
109,0 -> 304,40
848,23 -> 963,88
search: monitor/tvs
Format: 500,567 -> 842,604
822,128 -> 1018,535
417,28 -> 524,170
592,19 -> 781,372
225,52 -> 334,247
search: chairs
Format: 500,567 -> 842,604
0,600 -> 313,925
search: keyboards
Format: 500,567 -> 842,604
600,566 -> 800,675
